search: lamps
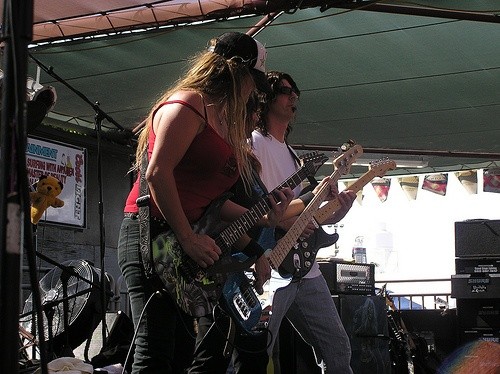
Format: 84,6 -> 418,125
323,157 -> 428,169
26,77 -> 56,131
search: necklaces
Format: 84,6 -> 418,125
215,106 -> 225,128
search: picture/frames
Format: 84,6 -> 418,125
26,133 -> 88,230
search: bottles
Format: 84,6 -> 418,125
353,236 -> 367,265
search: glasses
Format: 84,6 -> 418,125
273,85 -> 297,96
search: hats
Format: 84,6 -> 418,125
207,32 -> 273,95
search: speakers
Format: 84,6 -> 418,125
23,310 -> 133,364
331,294 -> 391,374
450,220 -> 500,345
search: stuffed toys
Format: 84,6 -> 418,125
29,174 -> 64,225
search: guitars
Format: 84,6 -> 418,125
137,149 -> 329,320
213,139 -> 364,337
269,157 -> 397,283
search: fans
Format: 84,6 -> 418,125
21,258 -> 110,357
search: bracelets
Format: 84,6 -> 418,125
241,239 -> 265,260
299,191 -> 315,207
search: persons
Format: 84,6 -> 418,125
118,32 -> 294,374
209,70 -> 353,374
